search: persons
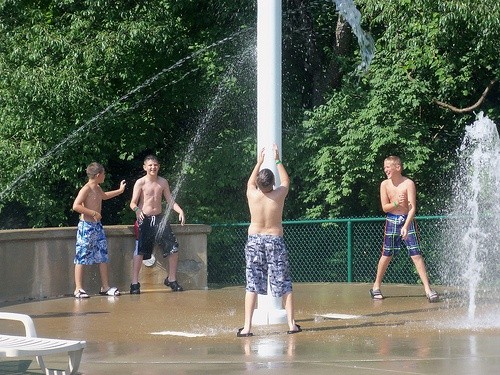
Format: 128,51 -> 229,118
130,155 -> 186,294
73,162 -> 126,298
370,156 -> 440,302
237,145 -> 302,337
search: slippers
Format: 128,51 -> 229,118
369,288 -> 384,299
427,292 -> 439,302
237,328 -> 253,336
287,323 -> 302,334
73,289 -> 89,298
99,287 -> 120,296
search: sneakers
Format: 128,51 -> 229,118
129,282 -> 140,294
164,276 -> 183,291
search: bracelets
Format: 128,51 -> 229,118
135,207 -> 138,212
276,161 -> 281,164
92,211 -> 96,216
394,201 -> 397,208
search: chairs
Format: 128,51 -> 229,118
0,312 -> 86,375
